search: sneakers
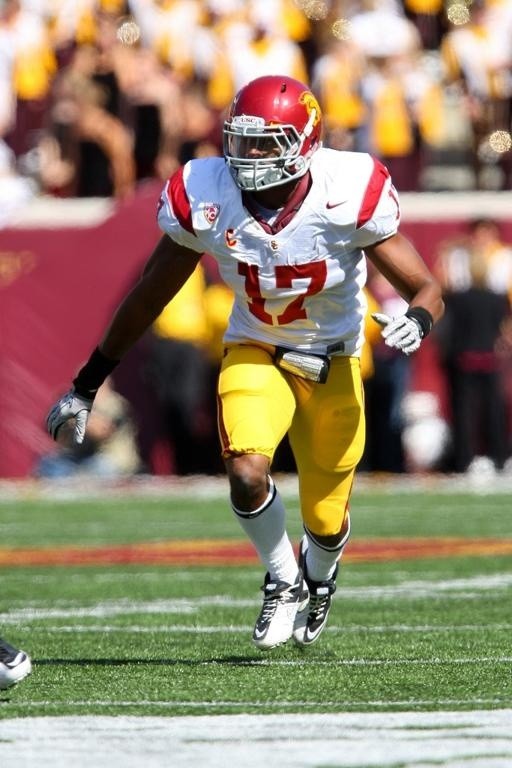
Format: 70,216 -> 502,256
0,639 -> 34,689
292,535 -> 339,648
252,568 -> 311,652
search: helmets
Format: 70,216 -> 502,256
220,75 -> 323,196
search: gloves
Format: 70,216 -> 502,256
45,386 -> 92,446
370,307 -> 434,357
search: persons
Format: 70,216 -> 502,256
38,218 -> 512,482
0,631 -> 32,689
39,75 -> 447,651
2,1 -> 512,202
